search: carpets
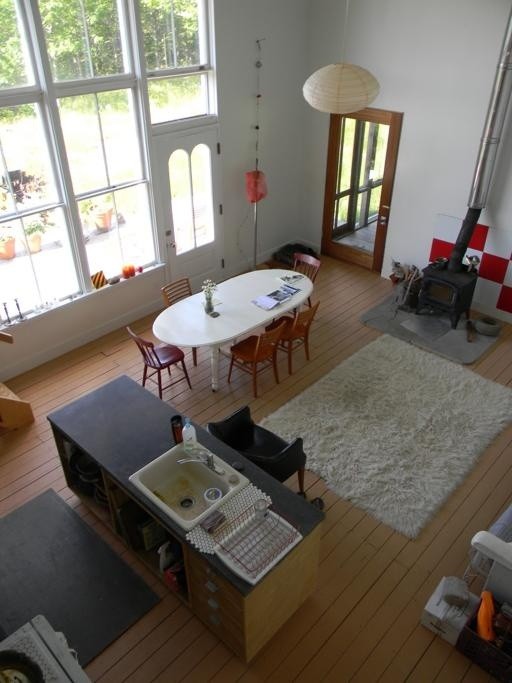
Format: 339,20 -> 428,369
0,486 -> 163,669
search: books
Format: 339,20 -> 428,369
252,288 -> 291,310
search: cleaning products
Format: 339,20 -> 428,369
156,540 -> 174,575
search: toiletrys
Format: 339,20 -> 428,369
182,417 -> 197,451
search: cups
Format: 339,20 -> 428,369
253,497 -> 268,521
169,414 -> 184,444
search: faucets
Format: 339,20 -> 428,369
177,447 -> 214,469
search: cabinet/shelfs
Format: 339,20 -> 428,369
187,547 -> 246,666
50,422 -> 117,535
100,468 -> 191,609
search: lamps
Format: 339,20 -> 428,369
301,0 -> 382,116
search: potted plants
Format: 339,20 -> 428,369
0,192 -> 116,260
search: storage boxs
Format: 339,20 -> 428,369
419,574 -> 511,683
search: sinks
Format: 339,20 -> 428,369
127,441 -> 250,532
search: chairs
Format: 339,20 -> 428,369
126,251 -> 323,400
205,404 -> 308,498
